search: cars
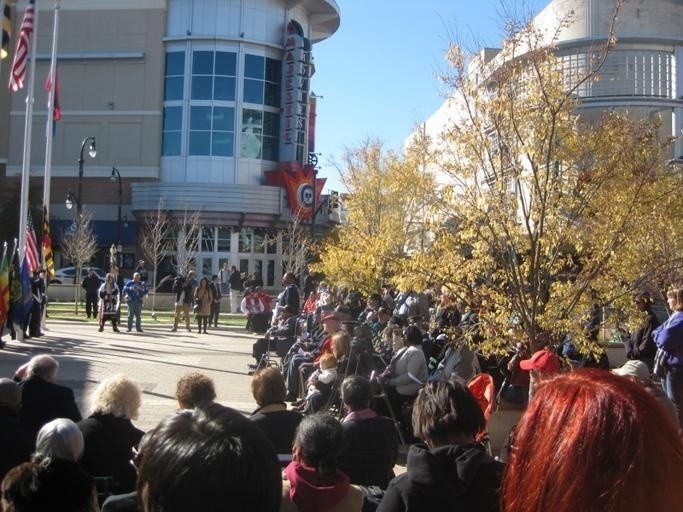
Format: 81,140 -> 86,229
49,264 -> 134,288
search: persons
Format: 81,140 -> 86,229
29,270 -> 45,337
614,292 -> 659,370
609,360 -> 677,432
82,260 -> 241,334
503,367 -> 682,512
242,268 -> 606,415
1,354 -> 503,512
650,285 -> 682,423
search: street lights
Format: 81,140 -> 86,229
66,135 -> 98,221
106,164 -> 122,274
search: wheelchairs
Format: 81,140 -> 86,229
251,316 -> 303,378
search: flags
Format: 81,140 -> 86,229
45,62 -> 60,137
0,201 -> 55,339
0,1 -> 11,60
7,0 -> 34,94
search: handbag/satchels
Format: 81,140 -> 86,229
193,298 -> 202,313
380,364 -> 394,378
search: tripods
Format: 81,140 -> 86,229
324,346 -> 409,455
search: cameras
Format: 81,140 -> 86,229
348,324 -> 372,346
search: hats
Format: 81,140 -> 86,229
520,350 -> 560,374
612,360 -> 650,379
321,314 -> 337,321
277,304 -> 294,314
